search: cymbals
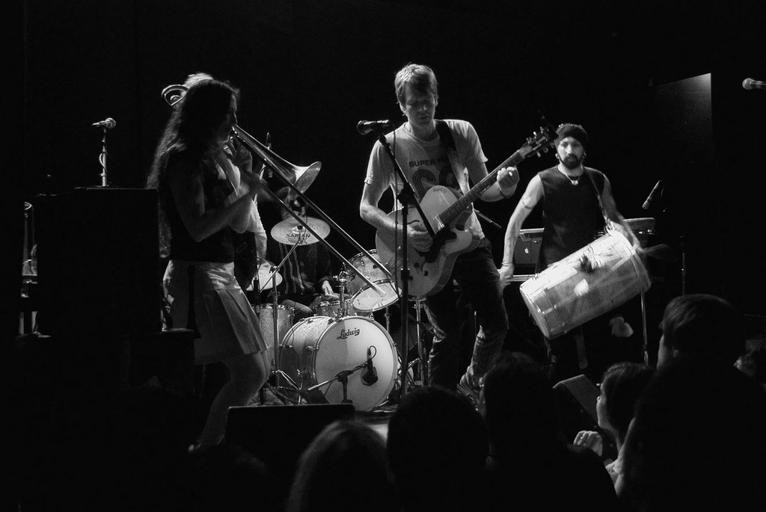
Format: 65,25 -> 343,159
271,215 -> 330,245
246,260 -> 282,291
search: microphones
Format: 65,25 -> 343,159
743,77 -> 766,90
91,118 -> 117,129
361,349 -> 378,386
357,119 -> 389,134
641,180 -> 660,210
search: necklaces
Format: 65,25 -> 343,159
562,167 -> 585,187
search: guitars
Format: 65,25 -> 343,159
375,127 -> 560,296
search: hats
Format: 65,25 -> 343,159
553,123 -> 587,148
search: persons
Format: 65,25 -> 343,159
291,295 -> 766,511
359,63 -> 523,409
2,294 -> 284,510
497,124 -> 645,385
160,71 -> 268,305
19,243 -> 42,335
144,77 -> 270,450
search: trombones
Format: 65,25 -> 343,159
161,83 -> 392,295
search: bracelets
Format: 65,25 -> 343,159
499,187 -> 511,199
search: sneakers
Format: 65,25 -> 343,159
459,372 -> 480,400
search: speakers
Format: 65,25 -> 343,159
225,404 -> 356,469
34,187 -> 162,338
553,373 -> 601,427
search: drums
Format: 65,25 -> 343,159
519,228 -> 651,341
342,250 -> 400,312
252,303 -> 295,373
311,299 -> 374,320
278,313 -> 398,412
